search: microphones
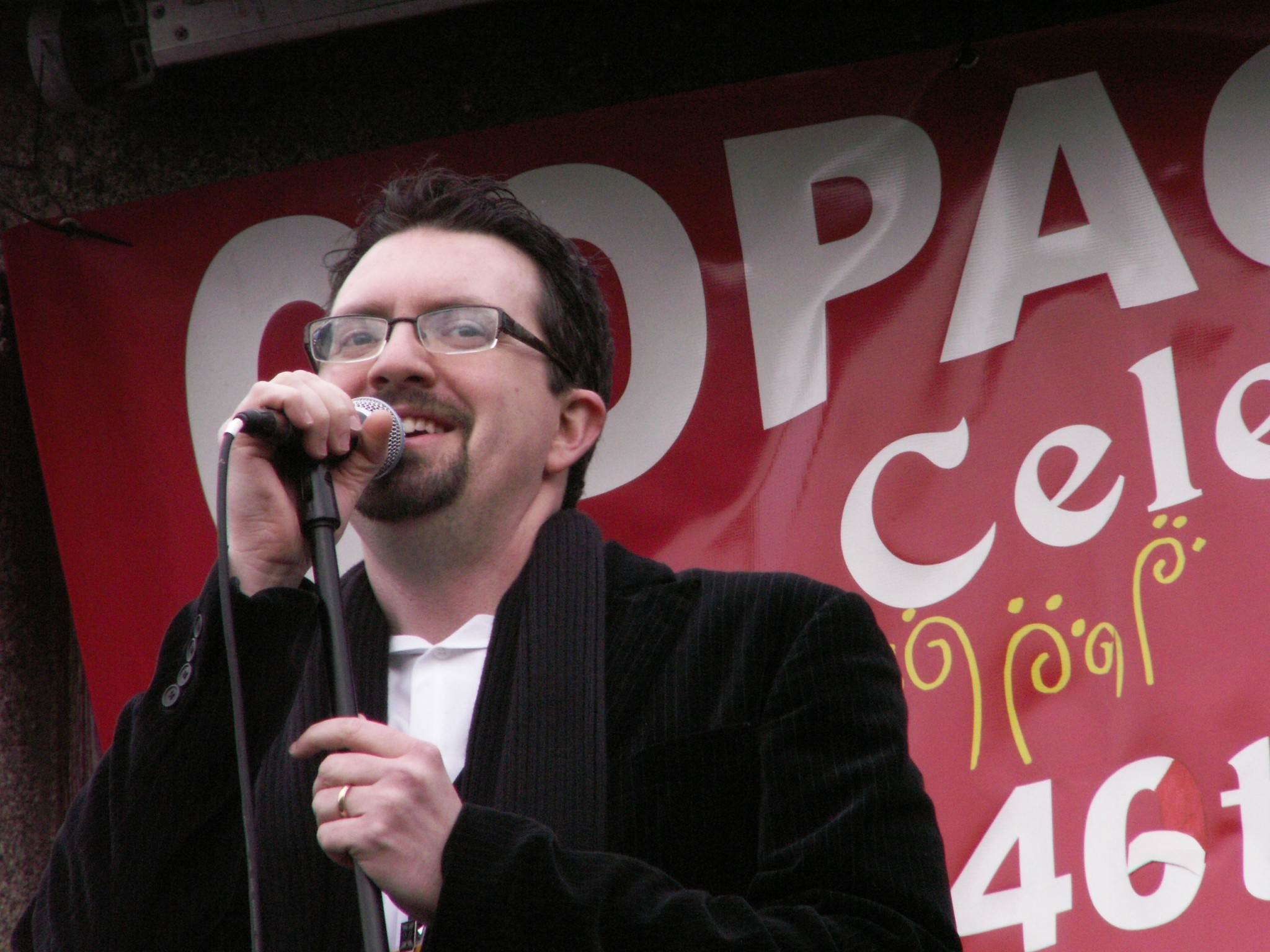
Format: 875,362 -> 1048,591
230,395 -> 405,481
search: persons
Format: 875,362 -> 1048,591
4,167 -> 965,952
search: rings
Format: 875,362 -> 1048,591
338,785 -> 352,820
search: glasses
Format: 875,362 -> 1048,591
309,304 -> 578,384
